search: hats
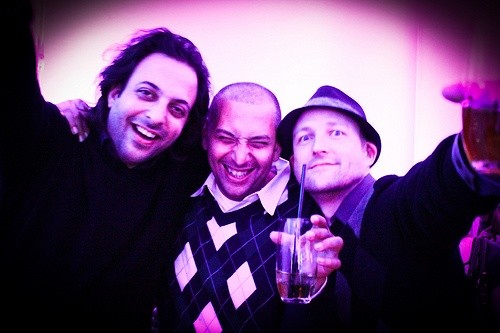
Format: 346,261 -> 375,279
276,85 -> 381,168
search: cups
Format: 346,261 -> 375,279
276,218 -> 317,305
461,45 -> 500,174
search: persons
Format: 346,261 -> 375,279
0,0 -> 210,333
152,82 -> 348,333
276,79 -> 500,333
